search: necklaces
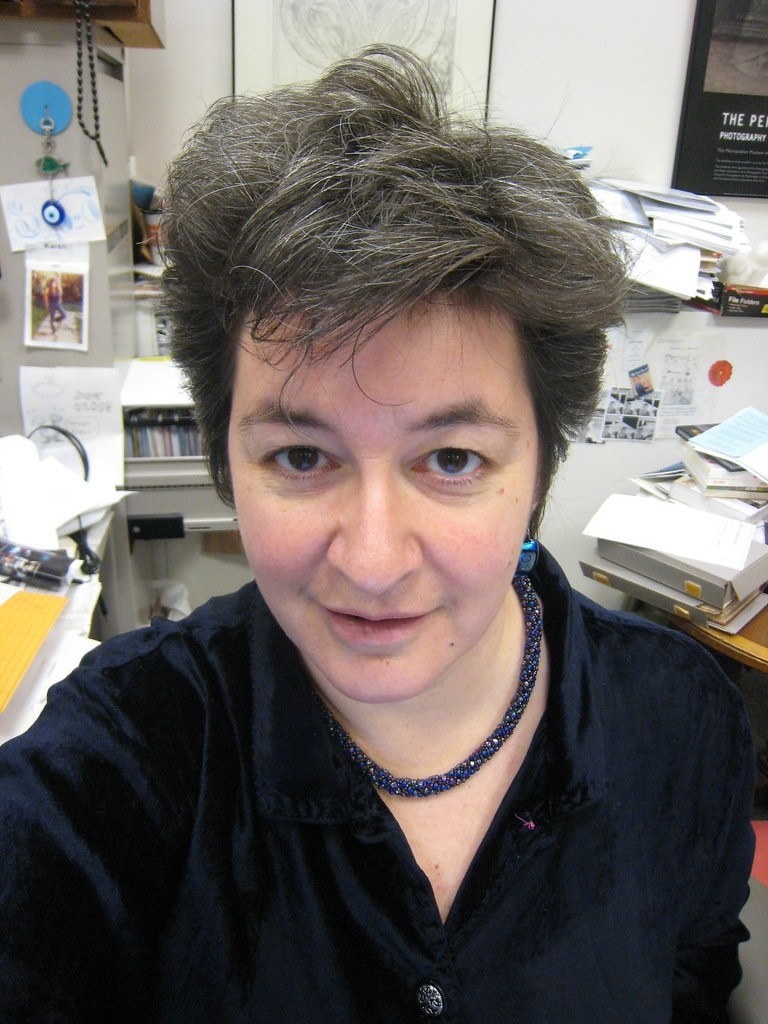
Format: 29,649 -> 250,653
317,572 -> 541,799
76,0 -> 110,165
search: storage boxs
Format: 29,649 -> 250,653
689,280 -> 768,318
578,538 -> 768,635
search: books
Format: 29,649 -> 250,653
671,424 -> 767,501
588,177 -> 749,313
127,424 -> 208,458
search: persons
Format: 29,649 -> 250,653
44,279 -> 67,336
0,44 -> 767,1023
